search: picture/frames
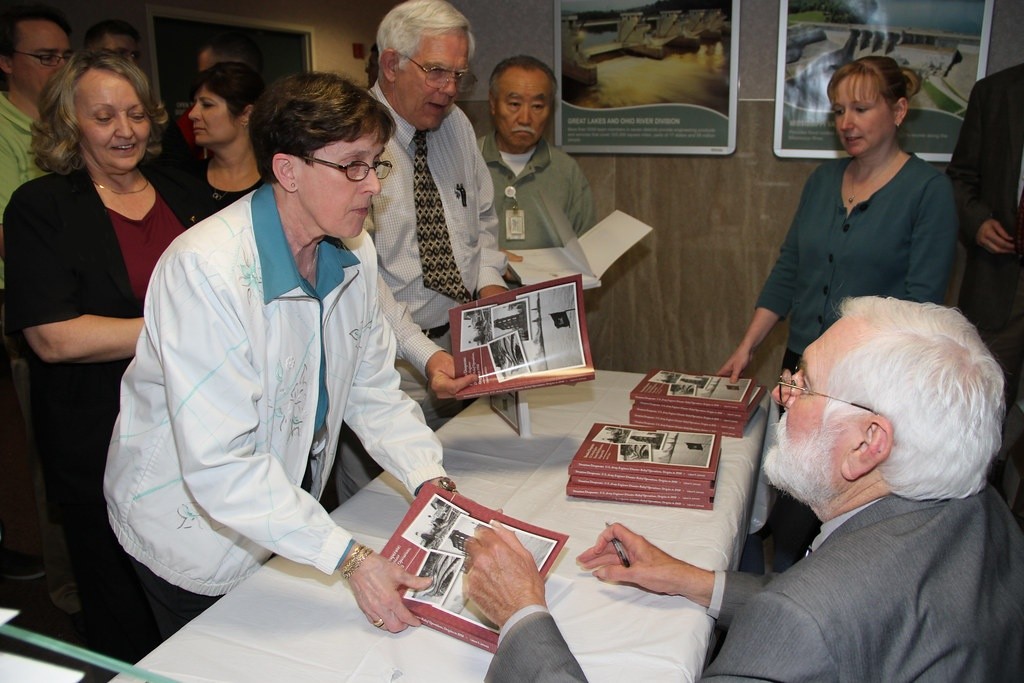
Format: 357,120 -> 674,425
551,0 -> 740,157
775,0 -> 995,165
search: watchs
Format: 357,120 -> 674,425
439,476 -> 457,492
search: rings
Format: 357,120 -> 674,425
374,619 -> 384,627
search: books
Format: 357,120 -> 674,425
630,368 -> 770,439
447,274 -> 596,401
567,422 -> 723,511
506,210 -> 653,289
374,481 -> 574,653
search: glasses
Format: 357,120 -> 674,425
776,369 -> 874,417
398,53 -> 479,94
303,155 -> 394,181
16,52 -> 74,66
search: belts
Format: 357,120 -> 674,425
422,322 -> 450,340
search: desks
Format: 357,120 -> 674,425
108,371 -> 772,683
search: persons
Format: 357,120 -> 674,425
465,295 -> 1024,683
331,1 -> 510,504
0,11 -> 276,666
711,54 -> 960,572
476,56 -> 599,290
947,65 -> 1024,514
104,72 -> 503,637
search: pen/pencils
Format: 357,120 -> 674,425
457,183 -> 467,207
605,521 -> 630,568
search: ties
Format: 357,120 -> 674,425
412,129 -> 472,305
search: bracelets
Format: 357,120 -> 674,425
341,546 -> 374,578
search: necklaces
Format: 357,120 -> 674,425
212,160 -> 244,200
304,244 -> 318,280
90,178 -> 148,194
